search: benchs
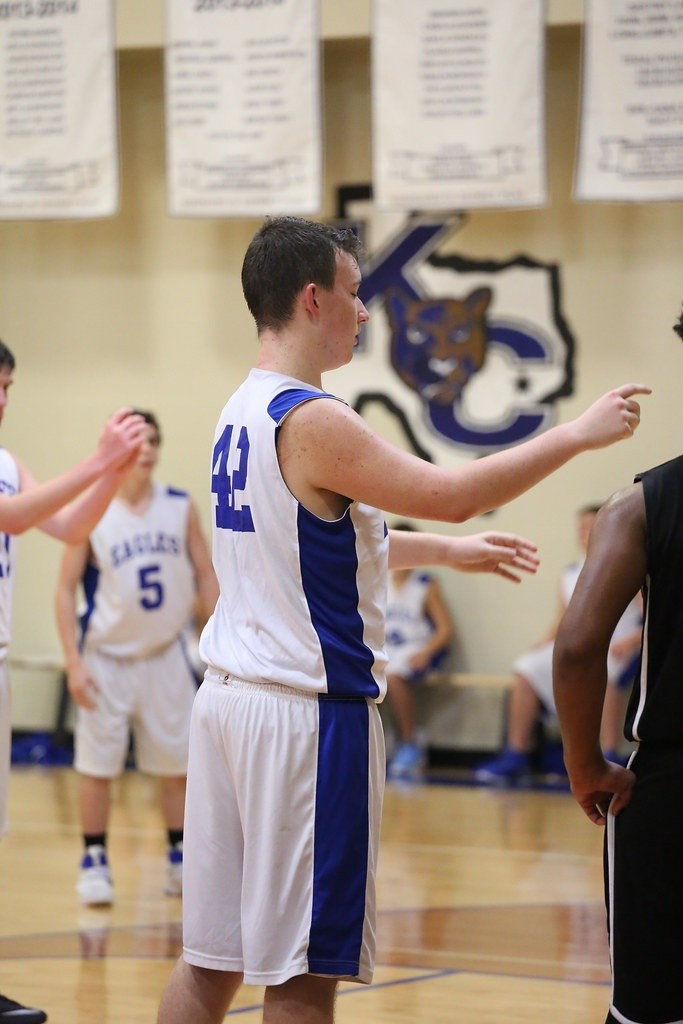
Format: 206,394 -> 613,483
411,668 -> 642,782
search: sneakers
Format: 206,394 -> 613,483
79,845 -> 113,903
168,840 -> 183,891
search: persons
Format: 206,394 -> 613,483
156,216 -> 652,1024
378,522 -> 456,780
475,503 -> 643,786
552,455 -> 683,1024
0,341 -> 156,1024
53,410 -> 220,905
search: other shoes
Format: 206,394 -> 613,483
389,743 -> 427,780
0,993 -> 47,1024
476,746 -> 538,784
547,751 -> 621,787
